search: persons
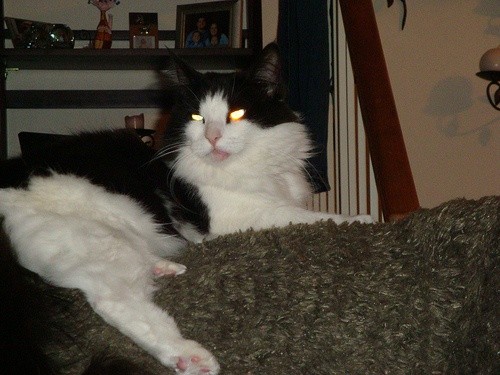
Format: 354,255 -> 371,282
185,16 -> 229,48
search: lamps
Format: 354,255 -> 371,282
475,47 -> 500,111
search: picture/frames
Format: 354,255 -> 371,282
174,0 -> 244,48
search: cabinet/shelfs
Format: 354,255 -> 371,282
0,0 -> 265,160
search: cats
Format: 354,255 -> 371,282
0,40 -> 375,375
